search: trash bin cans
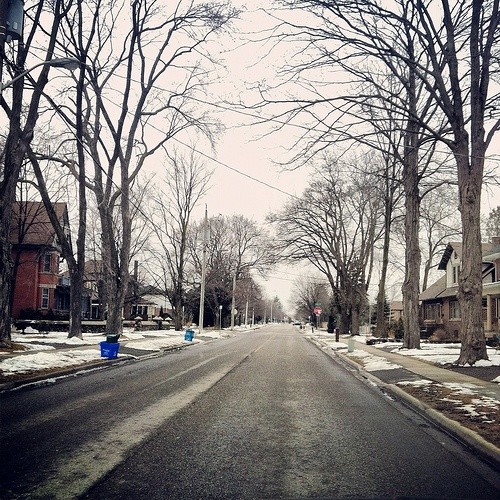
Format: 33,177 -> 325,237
100,332 -> 122,361
184,329 -> 196,343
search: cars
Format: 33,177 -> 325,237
293,322 -> 302,325
